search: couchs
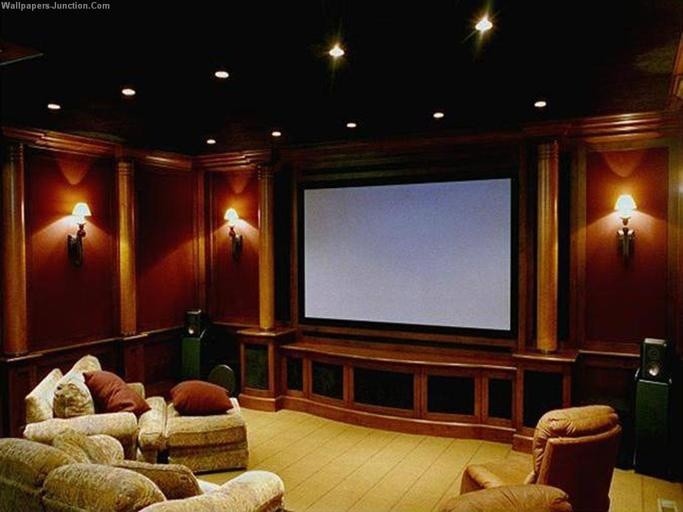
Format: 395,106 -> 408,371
0,435 -> 285,512
430,479 -> 573,510
461,404 -> 621,512
22,357 -> 252,473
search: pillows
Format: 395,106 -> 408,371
164,380 -> 232,415
118,459 -> 201,497
53,369 -> 91,415
80,370 -> 152,412
30,436 -> 124,465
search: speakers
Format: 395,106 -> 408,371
641,338 -> 667,383
186,309 -> 202,338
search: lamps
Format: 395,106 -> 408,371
64,200 -> 98,272
612,194 -> 640,269
223,205 -> 249,268
